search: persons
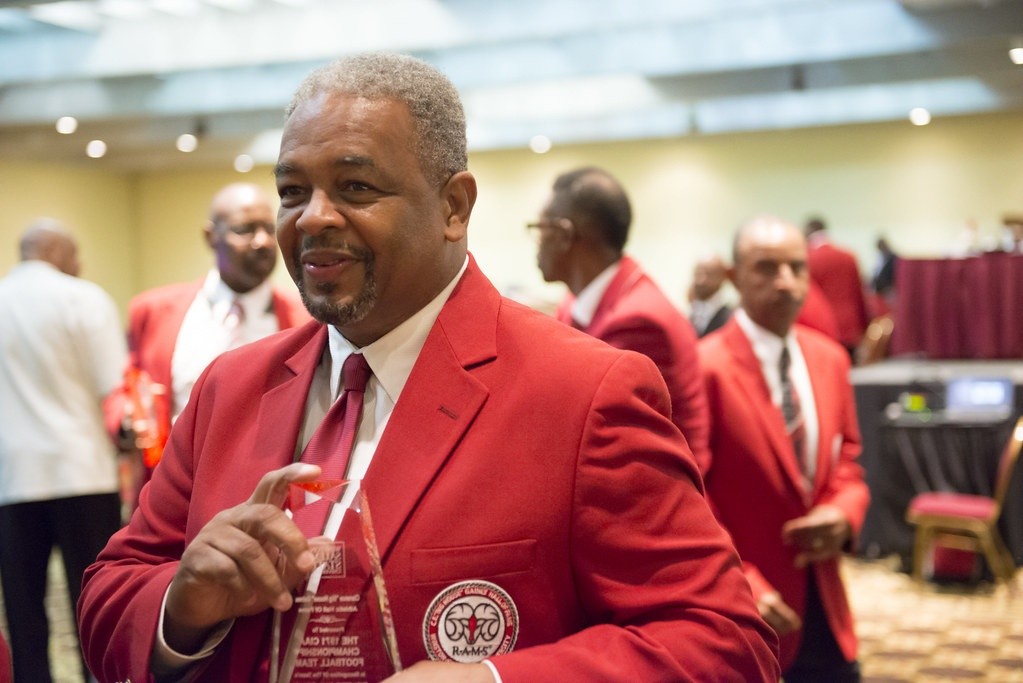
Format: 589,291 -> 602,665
74,52 -> 782,683
701,217 -> 868,683
102,185 -> 315,516
0,221 -> 128,683
535,169 -> 712,481
684,258 -> 734,339
795,219 -> 901,350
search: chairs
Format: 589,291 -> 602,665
904,419 -> 1023,587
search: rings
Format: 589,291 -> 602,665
814,540 -> 820,552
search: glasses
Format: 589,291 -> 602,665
524,221 -> 561,241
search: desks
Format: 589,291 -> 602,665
848,357 -> 1023,576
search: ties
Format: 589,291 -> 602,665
250,353 -> 371,683
776,350 -> 810,480
226,304 -> 245,351
